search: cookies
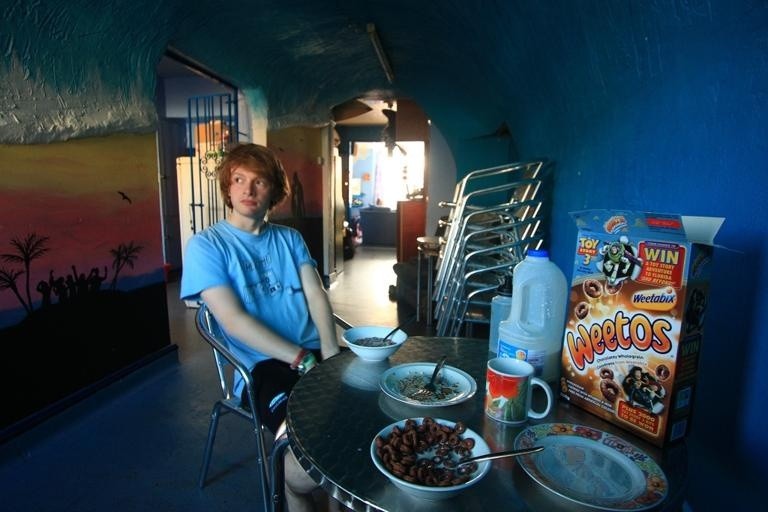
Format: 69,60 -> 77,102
375,416 -> 477,487
574,279 -> 669,403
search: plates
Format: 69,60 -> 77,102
378,362 -> 477,407
370,418 -> 492,493
515,423 -> 669,511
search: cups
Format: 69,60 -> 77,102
484,358 -> 554,424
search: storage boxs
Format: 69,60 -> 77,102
194,122 -> 231,143
194,143 -> 229,159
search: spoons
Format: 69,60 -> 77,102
371,315 -> 417,346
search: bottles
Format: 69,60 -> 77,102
489,276 -> 513,353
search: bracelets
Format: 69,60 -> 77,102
297,351 -> 317,377
291,349 -> 308,369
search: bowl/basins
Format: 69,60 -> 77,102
341,326 -> 407,361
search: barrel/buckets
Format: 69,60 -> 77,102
497,248 -> 569,384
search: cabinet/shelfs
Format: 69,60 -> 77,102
397,200 -> 426,264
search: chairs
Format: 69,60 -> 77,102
194,301 -> 354,511
416,157 -> 546,337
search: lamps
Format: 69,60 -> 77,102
381,99 -> 406,158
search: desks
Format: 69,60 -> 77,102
270,337 -> 688,512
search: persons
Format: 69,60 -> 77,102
178,144 -> 351,512
